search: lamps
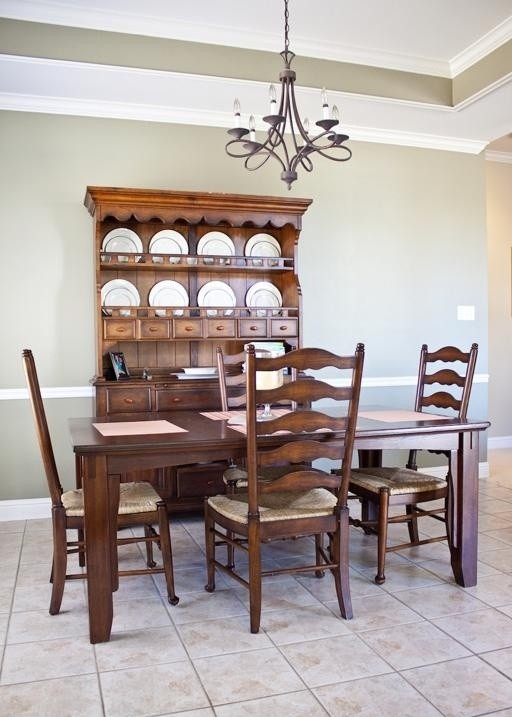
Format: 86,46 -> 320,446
224,0 -> 351,192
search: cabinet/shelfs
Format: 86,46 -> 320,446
90,380 -> 313,519
83,187 -> 313,383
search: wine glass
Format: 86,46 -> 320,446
253,346 -> 282,422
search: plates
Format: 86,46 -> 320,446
100,228 -> 283,317
182,367 -> 218,374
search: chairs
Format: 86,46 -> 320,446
214,345 -> 337,572
22,348 -> 179,615
329,342 -> 479,585
205,342 -> 365,634
